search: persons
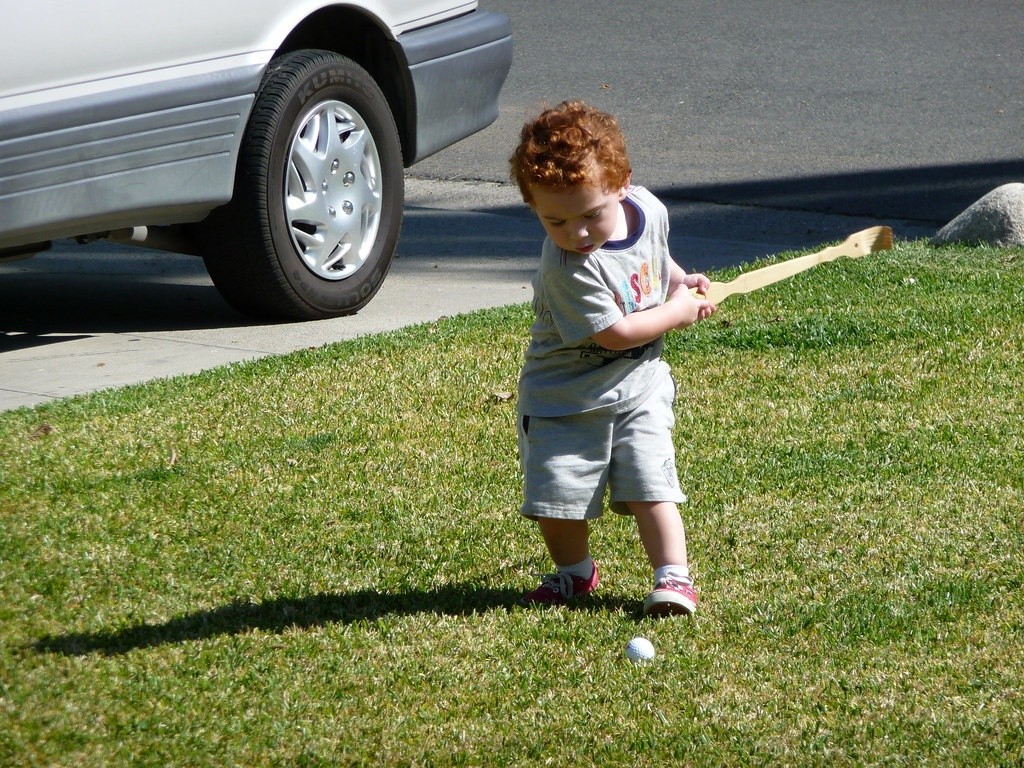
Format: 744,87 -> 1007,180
508,98 -> 716,617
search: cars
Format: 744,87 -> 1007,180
1,1 -> 515,325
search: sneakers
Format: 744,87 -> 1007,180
522,561 -> 599,602
646,572 -> 697,614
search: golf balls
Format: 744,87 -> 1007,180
625,637 -> 655,662
